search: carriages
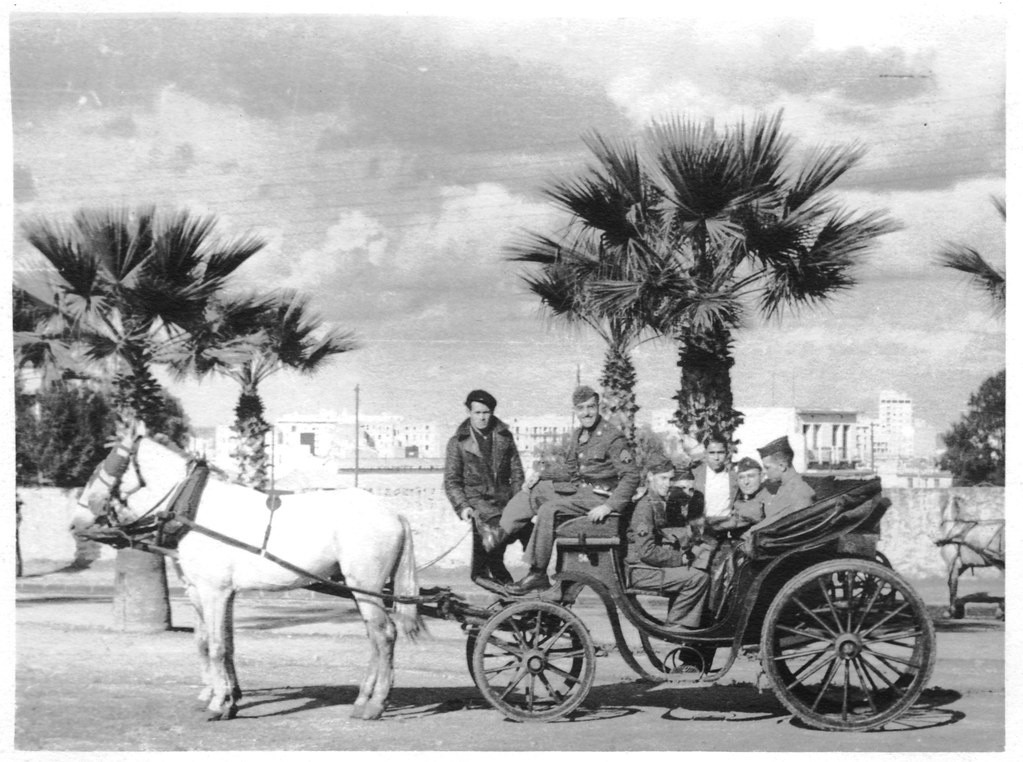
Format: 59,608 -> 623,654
71,411 -> 939,730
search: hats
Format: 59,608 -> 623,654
757,435 -> 789,458
573,385 -> 595,404
737,457 -> 761,473
469,390 -> 498,414
671,467 -> 694,481
651,458 -> 674,474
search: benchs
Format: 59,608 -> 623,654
742,474 -> 892,557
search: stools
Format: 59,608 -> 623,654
551,513 -> 621,545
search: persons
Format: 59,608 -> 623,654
471,386 -> 639,594
709,436 -> 817,621
692,436 -> 739,518
625,454 -> 709,630
733,457 -> 771,523
444,390 -> 534,583
666,471 -> 694,524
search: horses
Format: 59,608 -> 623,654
68,406 -> 433,723
934,494 -> 1005,622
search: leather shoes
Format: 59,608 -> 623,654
475,511 -> 509,553
506,569 -> 550,595
